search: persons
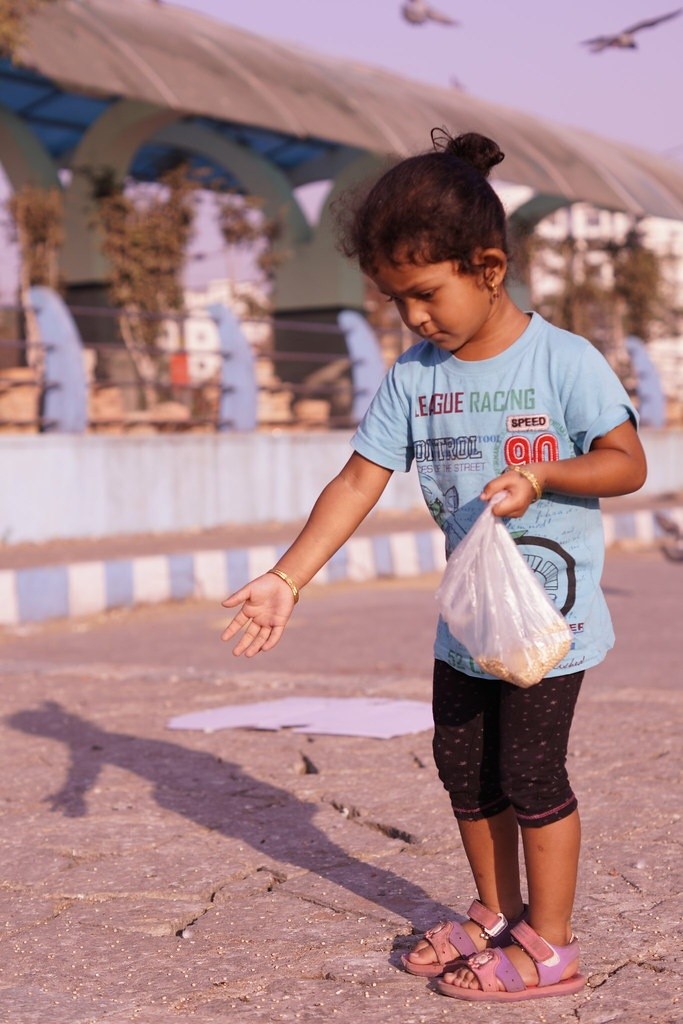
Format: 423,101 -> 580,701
220,131 -> 646,1001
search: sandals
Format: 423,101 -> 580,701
437,920 -> 584,1001
400,899 -> 532,977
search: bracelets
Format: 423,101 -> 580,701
268,570 -> 299,604
502,466 -> 541,504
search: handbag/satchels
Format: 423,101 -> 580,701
435,490 -> 574,688
